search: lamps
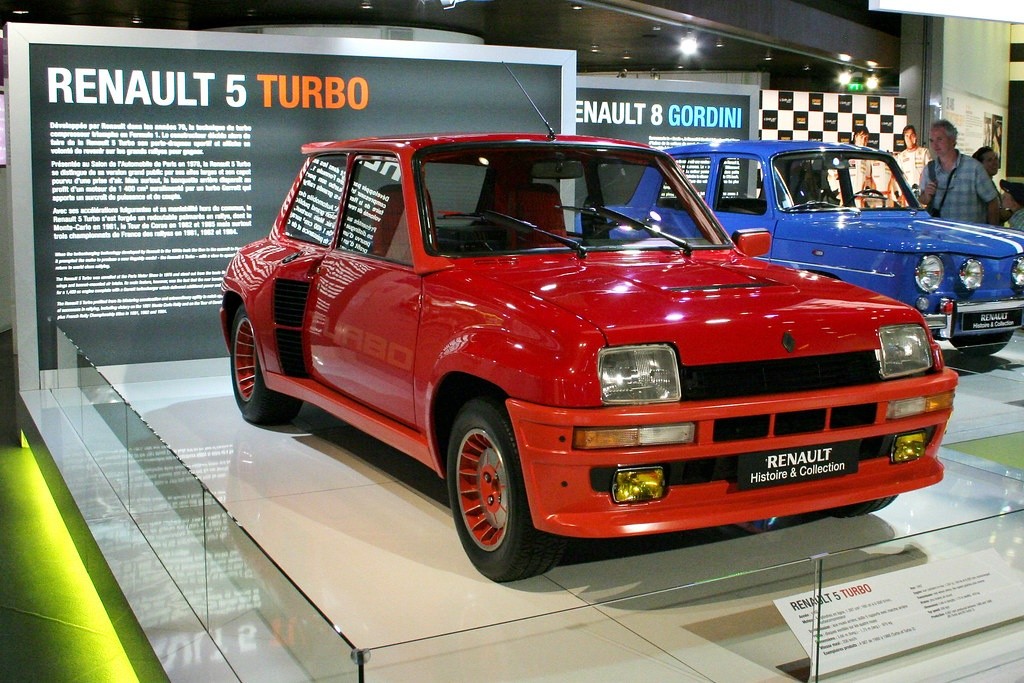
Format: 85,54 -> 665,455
617,69 -> 628,79
802,62 -> 813,71
713,36 -> 728,48
761,48 -> 776,61
650,67 -> 661,80
679,27 -> 698,56
589,40 -> 601,53
622,47 -> 634,60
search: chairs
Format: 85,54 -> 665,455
499,183 -> 576,245
366,180 -> 439,263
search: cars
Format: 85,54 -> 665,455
220,129 -> 962,584
573,139 -> 1024,355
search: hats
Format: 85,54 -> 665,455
1000,179 -> 1024,207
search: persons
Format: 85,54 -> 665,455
890,126 -> 932,207
918,121 -> 1000,227
972,146 -> 1024,233
829,128 -> 876,209
